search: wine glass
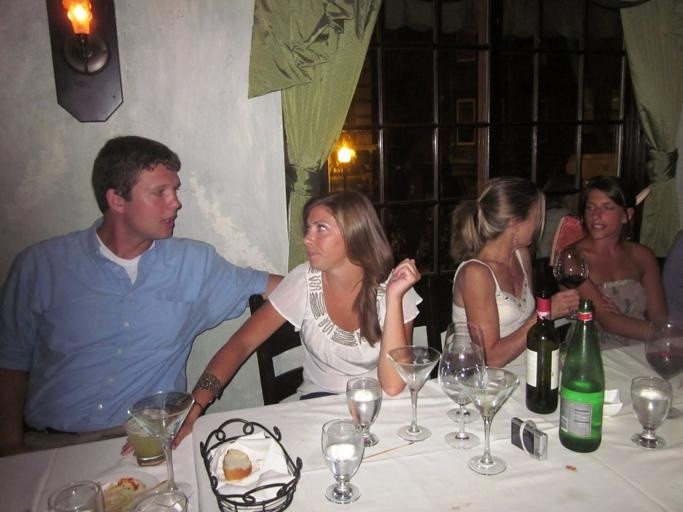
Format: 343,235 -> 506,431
345,376 -> 383,446
388,345 -> 440,441
631,375 -> 673,449
554,243 -> 591,323
642,317 -> 682,418
320,418 -> 364,506
456,365 -> 519,475
127,393 -> 195,501
436,323 -> 486,449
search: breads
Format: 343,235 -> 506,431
222,449 -> 253,481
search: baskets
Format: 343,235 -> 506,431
199,418 -> 302,512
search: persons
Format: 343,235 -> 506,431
446,174 -> 582,372
115,186 -> 424,459
556,172 -> 668,353
661,229 -> 683,337
0,135 -> 289,460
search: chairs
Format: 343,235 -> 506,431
424,273 -> 453,377
532,258 -> 571,343
249,295 -> 304,405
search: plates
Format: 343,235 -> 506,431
601,389 -> 622,416
92,469 -> 160,511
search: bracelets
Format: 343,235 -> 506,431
193,398 -> 206,416
193,372 -> 222,403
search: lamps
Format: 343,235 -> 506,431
44,1 -> 125,76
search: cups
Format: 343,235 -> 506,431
124,413 -> 166,468
45,482 -> 105,511
135,492 -> 190,512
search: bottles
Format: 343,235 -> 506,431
525,288 -> 559,414
558,299 -> 606,453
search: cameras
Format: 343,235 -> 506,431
510,417 -> 548,463
